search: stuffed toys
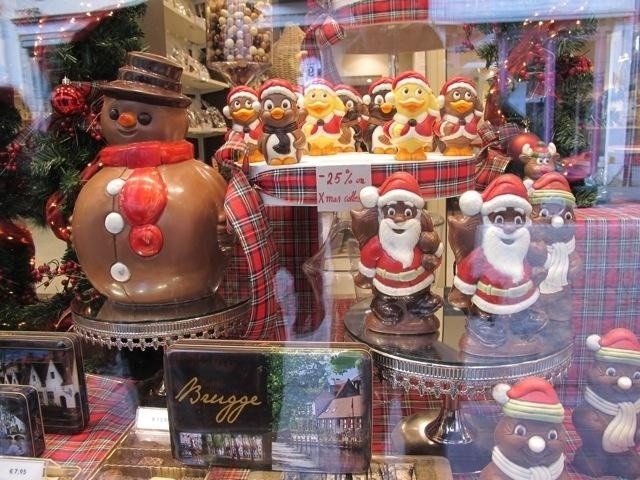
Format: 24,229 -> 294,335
479,376 -> 572,479
571,328 -> 640,478
214,72 -> 579,355
